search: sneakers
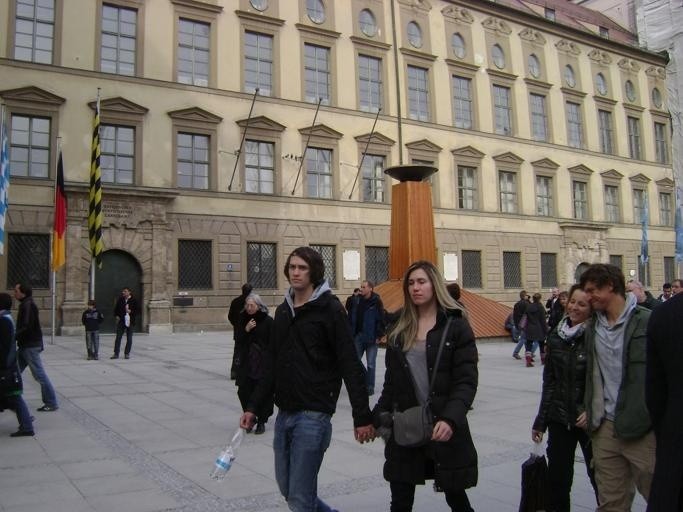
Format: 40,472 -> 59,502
86,353 -> 101,361
512,352 -> 522,360
10,430 -> 36,438
365,387 -> 376,396
526,352 -> 536,358
124,353 -> 130,359
110,354 -> 119,360
37,403 -> 60,412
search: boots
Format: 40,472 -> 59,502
541,351 -> 547,365
526,351 -> 535,368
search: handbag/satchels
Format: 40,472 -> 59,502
391,402 -> 431,447
0,364 -> 23,400
517,306 -> 531,330
517,436 -> 547,512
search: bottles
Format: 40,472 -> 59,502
208,424 -> 246,481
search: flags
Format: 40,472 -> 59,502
50,139 -> 66,273
0,105 -> 11,255
87,102 -> 103,271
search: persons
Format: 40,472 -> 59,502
0,292 -> 35,436
110,287 -> 138,359
346,261 -> 479,512
229,247 -> 376,512
81,300 -> 104,360
13,281 -> 60,411
505,263 -> 682,512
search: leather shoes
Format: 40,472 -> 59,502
245,417 -> 258,434
255,424 -> 266,433
